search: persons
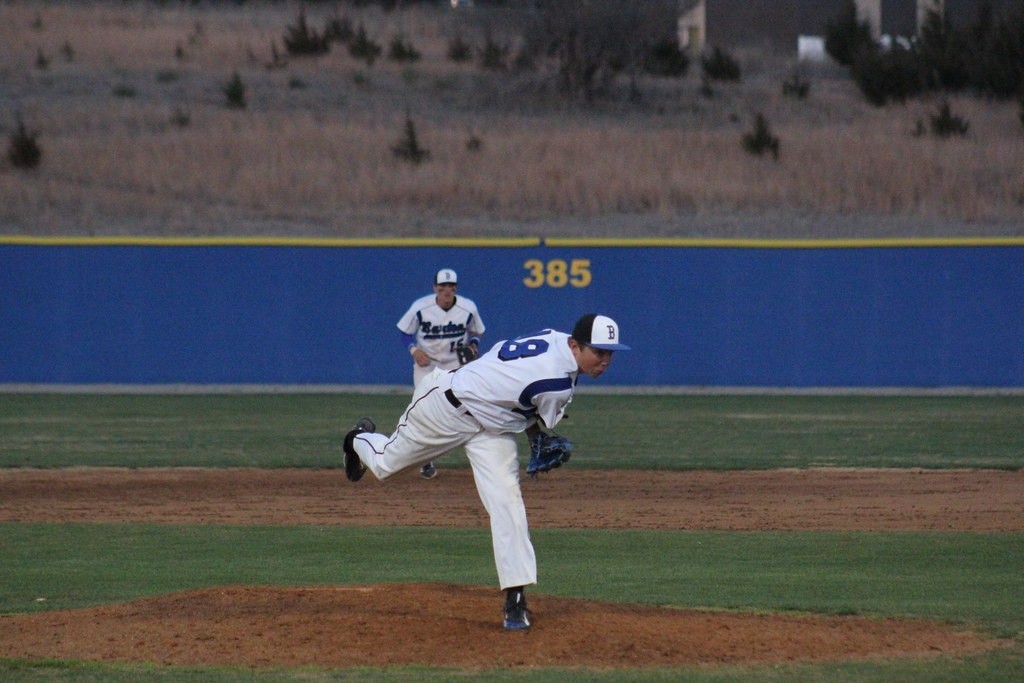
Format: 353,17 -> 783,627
396,267 -> 485,478
343,314 -> 632,631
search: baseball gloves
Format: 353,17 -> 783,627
525,431 -> 576,479
456,337 -> 481,366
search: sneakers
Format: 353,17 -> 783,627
420,462 -> 436,479
502,591 -> 531,630
344,418 -> 375,481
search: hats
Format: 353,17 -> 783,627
572,313 -> 632,351
434,268 -> 459,285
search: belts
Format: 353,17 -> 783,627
444,367 -> 472,416
